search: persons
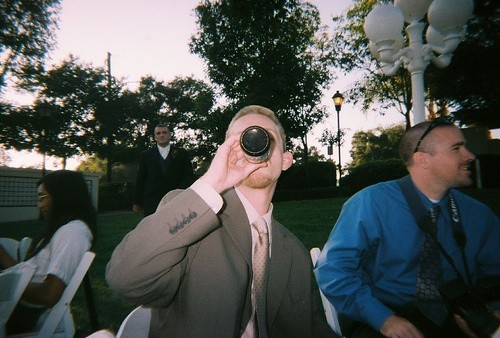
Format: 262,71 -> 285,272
0,170 -> 97,335
106,104 -> 339,338
312,121 -> 500,338
131,122 -> 196,221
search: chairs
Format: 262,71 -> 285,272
0,237 -> 341,338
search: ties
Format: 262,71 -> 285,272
252,218 -> 268,338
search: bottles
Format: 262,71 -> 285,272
239,125 -> 271,165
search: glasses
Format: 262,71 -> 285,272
37,193 -> 50,201
415,115 -> 455,152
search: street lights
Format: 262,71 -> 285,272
332,91 -> 344,178
